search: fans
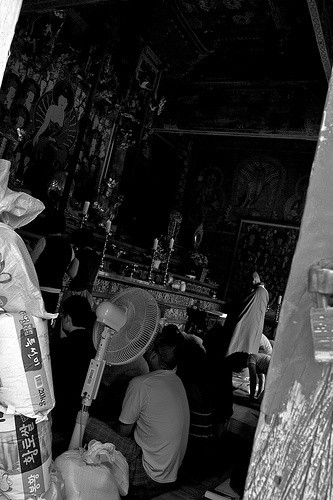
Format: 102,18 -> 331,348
68,287 -> 161,451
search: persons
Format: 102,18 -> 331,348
28,209 -> 274,500
91,179 -> 124,227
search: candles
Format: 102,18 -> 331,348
106,220 -> 112,232
83,201 -> 90,214
154,238 -> 158,251
169,238 -> 174,249
